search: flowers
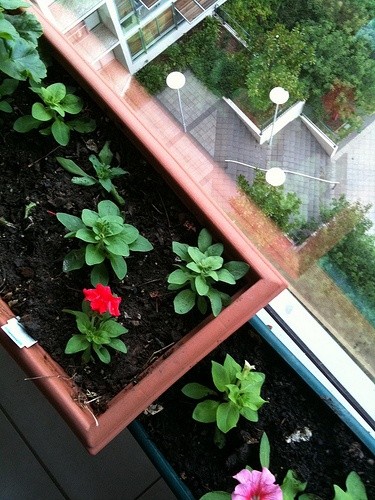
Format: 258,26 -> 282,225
58,282 -> 136,365
229,467 -> 282,500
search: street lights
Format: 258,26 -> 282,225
267,87 -> 290,170
166,71 -> 187,133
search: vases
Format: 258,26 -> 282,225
0,0 -> 294,457
128,313 -> 375,500
